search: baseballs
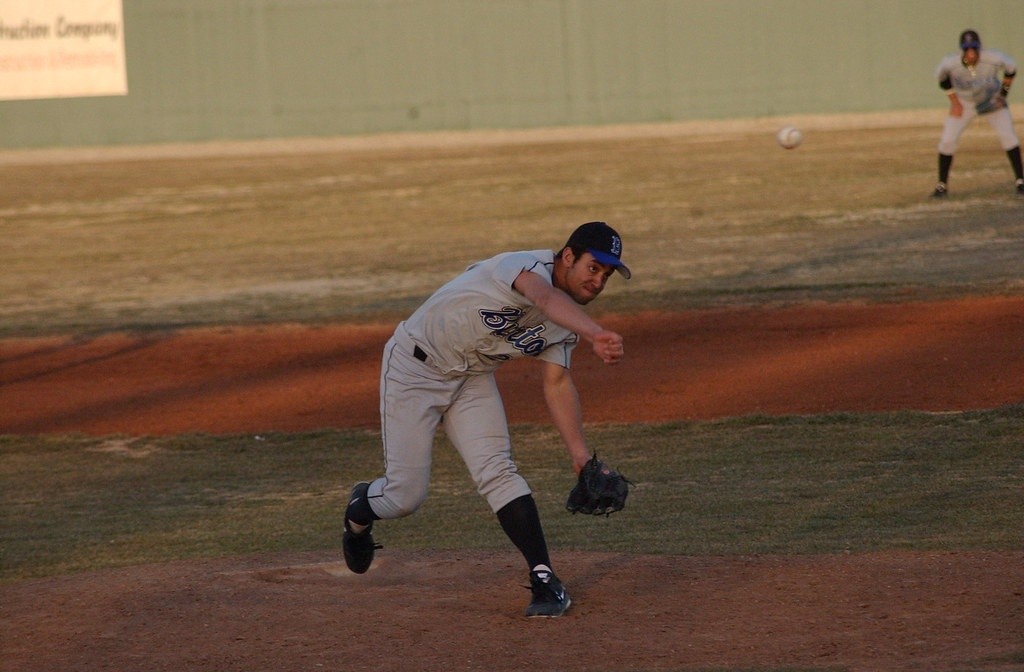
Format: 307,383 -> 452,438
778,125 -> 803,150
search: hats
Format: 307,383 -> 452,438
961,30 -> 980,49
566,222 -> 632,280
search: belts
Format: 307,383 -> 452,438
414,345 -> 427,362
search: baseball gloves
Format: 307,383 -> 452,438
563,447 -> 637,519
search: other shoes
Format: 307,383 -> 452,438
1015,177 -> 1024,192
932,187 -> 946,198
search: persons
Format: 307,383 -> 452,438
928,30 -> 1024,201
342,221 -> 632,618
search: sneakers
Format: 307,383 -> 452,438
525,573 -> 571,618
341,481 -> 384,574
